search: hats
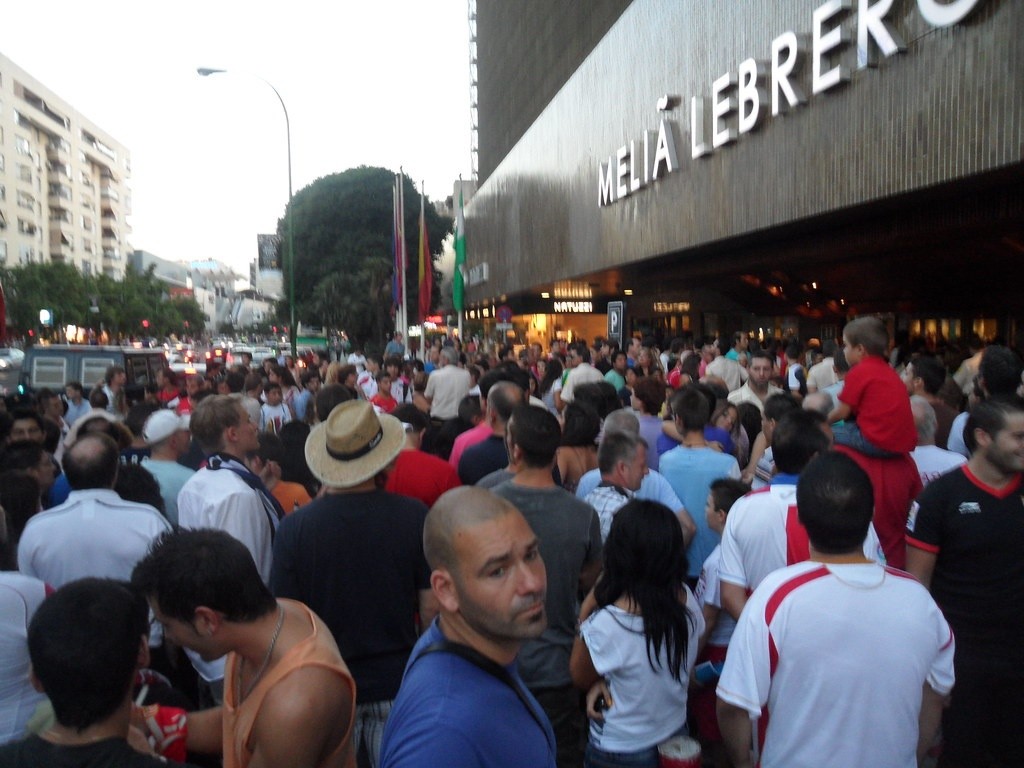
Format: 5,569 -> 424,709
141,408 -> 190,446
304,399 -> 406,488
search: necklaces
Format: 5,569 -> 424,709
238,602 -> 284,710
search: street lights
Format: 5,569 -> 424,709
197,68 -> 296,359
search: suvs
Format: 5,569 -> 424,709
20,346 -> 172,402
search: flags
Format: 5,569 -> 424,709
452,187 -> 466,312
392,186 -> 410,318
418,204 -> 433,324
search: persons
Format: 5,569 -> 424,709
0,317 -> 1024,706
489,405 -> 603,768
569,498 -> 706,768
695,478 -> 753,741
268,399 -> 430,768
716,449 -> 956,768
381,485 -> 557,768
127,525 -> 356,768
0,505 -> 56,744
903,393 -> 1024,768
0,576 -> 182,768
716,409 -> 888,624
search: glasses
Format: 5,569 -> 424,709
568,352 -> 577,360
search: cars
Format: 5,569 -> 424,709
164,339 -> 290,373
0,347 -> 25,372
